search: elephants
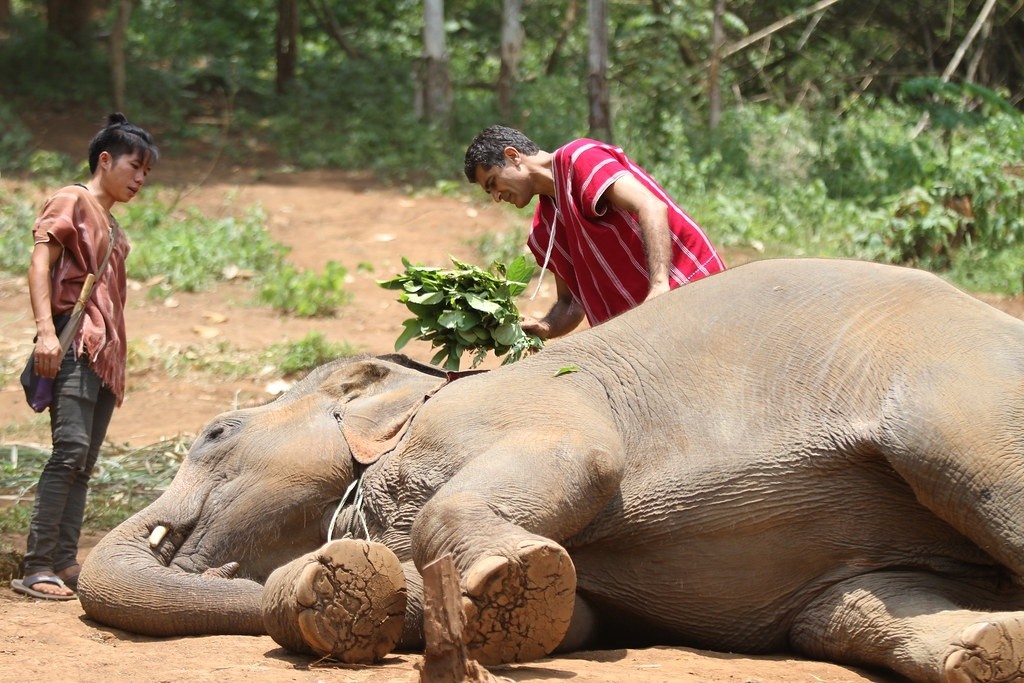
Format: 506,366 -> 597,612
75,259 -> 1024,683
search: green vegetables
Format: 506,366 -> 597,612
375,256 -> 546,372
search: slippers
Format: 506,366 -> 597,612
11,574 -> 79,600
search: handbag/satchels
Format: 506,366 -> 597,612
20,345 -> 55,413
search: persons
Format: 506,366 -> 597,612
465,126 -> 726,346
11,112 -> 161,601
